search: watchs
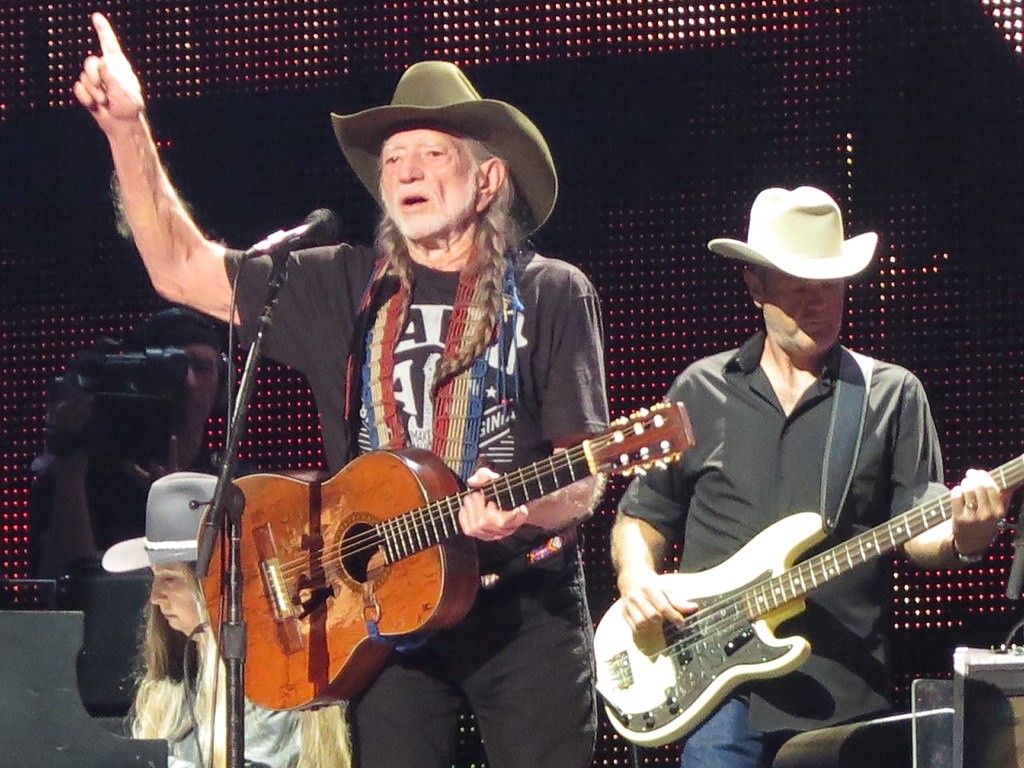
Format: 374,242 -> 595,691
952,537 -> 986,565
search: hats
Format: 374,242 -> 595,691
707,186 -> 879,279
102,472 -> 219,574
330,61 -> 559,240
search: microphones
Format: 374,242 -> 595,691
243,208 -> 340,260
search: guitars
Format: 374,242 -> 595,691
596,454 -> 1024,748
195,400 -> 697,713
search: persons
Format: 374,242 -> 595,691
609,178 -> 1011,768
46,4 -> 612,768
